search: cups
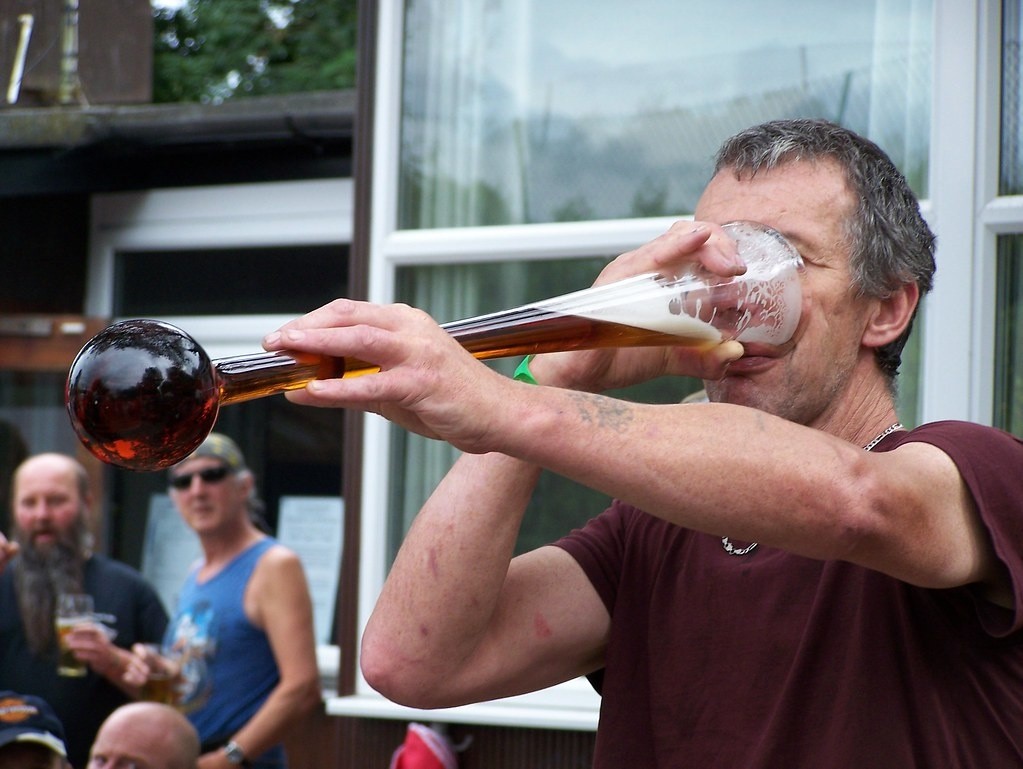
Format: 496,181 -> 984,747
65,221 -> 812,471
142,656 -> 180,705
54,594 -> 94,677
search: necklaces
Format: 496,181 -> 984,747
722,423 -> 903,554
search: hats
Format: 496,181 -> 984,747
0,690 -> 68,757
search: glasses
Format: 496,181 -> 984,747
171,467 -> 232,489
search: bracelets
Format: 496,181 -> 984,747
513,355 -> 539,385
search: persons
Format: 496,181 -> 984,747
0,452 -> 170,769
86,701 -> 201,769
262,115 -> 1023,769
0,688 -> 72,769
123,433 -> 322,769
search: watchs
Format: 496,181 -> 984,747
223,740 -> 250,769
